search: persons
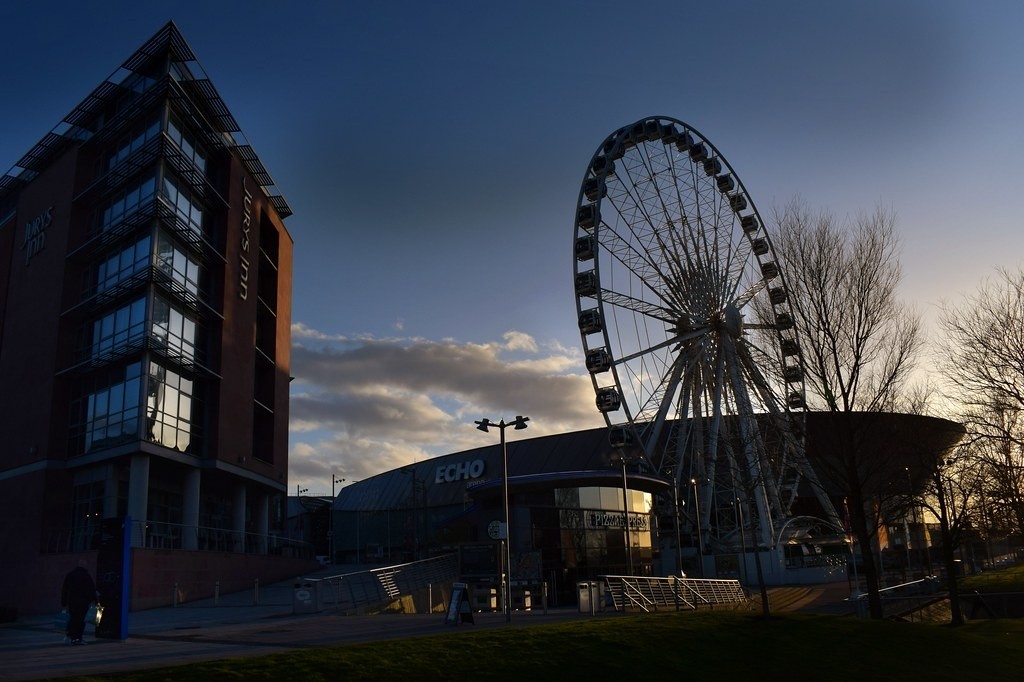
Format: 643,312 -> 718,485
61,559 -> 96,646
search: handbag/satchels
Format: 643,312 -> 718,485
84,599 -> 104,627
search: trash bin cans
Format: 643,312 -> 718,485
577,580 -> 598,615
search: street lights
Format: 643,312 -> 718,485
730,498 -> 752,598
611,456 -> 644,582
691,478 -> 705,578
474,415 -> 530,625
332,473 -> 346,569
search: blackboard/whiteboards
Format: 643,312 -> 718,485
444,587 -> 474,622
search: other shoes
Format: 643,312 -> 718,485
65,631 -> 70,640
71,639 -> 88,646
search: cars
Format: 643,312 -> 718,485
986,556 -> 1016,569
